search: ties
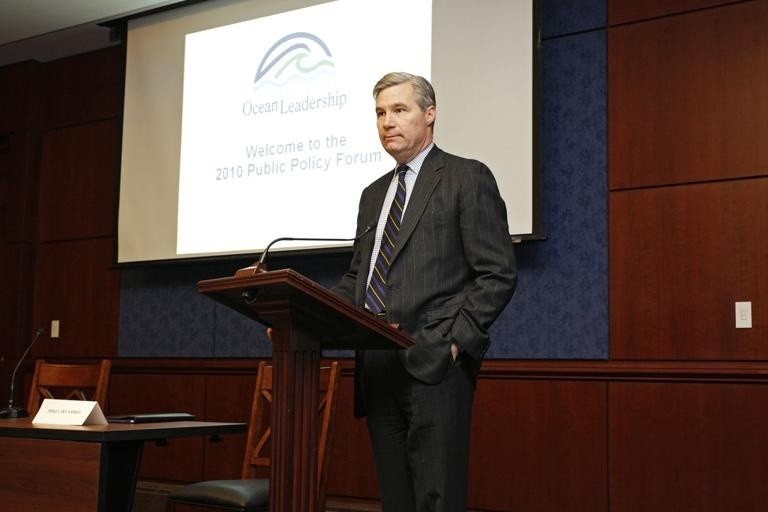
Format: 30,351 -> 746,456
365,164 -> 409,315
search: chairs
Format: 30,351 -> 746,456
164,360 -> 342,512
26,359 -> 113,416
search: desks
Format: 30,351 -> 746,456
0,416 -> 248,512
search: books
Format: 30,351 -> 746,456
105,411 -> 197,423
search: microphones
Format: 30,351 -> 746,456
234,222 -> 377,276
1,327 -> 46,419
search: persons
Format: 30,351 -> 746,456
265,71 -> 517,512
